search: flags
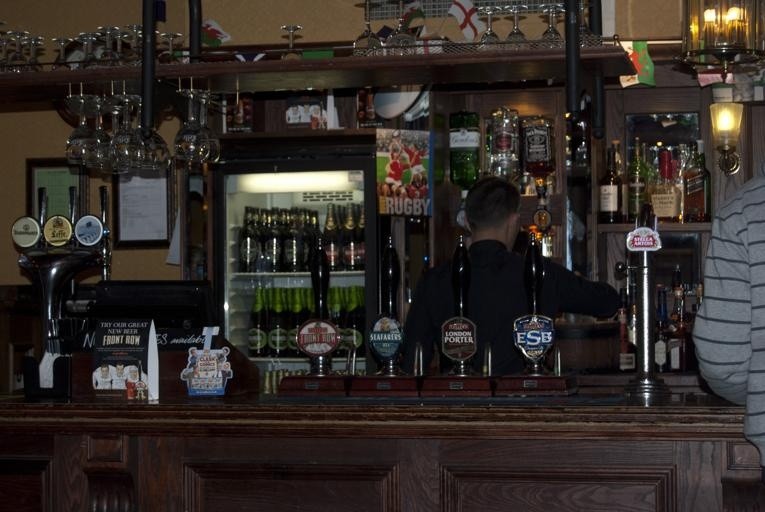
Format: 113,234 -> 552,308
615,39 -> 657,90
186,20 -> 230,49
448,0 -> 487,42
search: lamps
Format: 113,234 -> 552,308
709,102 -> 744,177
680,1 -> 763,84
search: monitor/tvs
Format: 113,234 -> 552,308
93,279 -> 215,350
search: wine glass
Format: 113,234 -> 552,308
577,2 -> 602,47
280,23 -> 305,60
0,22 -> 183,73
475,4 -> 564,50
351,0 -> 415,56
63,93 -> 222,176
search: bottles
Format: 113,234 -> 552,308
241,200 -> 365,272
599,136 -> 712,222
521,114 -> 554,198
449,112 -> 481,197
250,286 -> 367,356
488,107 -> 521,184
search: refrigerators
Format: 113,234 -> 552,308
216,133 -> 379,394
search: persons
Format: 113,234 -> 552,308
308,106 -> 324,122
124,364 -> 141,389
300,103 -> 313,124
214,361 -> 232,388
376,128 -> 430,200
215,346 -> 230,363
186,347 -> 199,369
181,364 -> 201,392
690,169 -> 765,469
394,175 -> 620,386
286,104 -> 301,124
92,364 -> 113,390
110,361 -> 127,390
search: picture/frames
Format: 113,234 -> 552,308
25,157 -> 90,236
113,172 -> 171,250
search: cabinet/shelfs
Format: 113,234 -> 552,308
379,85 -> 751,387
